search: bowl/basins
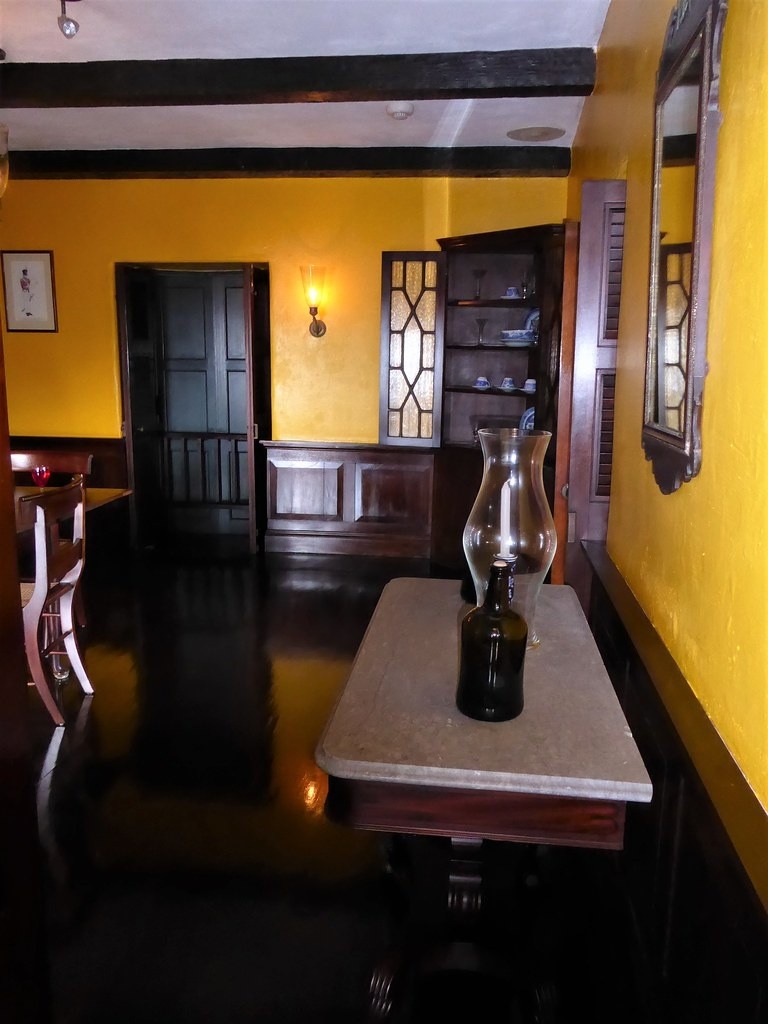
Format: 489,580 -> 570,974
499,330 -> 534,347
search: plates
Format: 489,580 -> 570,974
472,386 -> 492,391
520,389 -> 536,393
523,308 -> 540,345
501,296 -> 520,299
519,407 -> 535,430
497,387 -> 517,392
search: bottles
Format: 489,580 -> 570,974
456,563 -> 528,723
463,427 -> 556,651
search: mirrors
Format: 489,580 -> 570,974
640,0 -> 730,496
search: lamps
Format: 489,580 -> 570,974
301,265 -> 326,337
57,0 -> 82,39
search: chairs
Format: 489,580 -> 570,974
19,474 -> 95,727
10,451 -> 94,627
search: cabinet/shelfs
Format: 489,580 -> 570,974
436,223 -> 566,449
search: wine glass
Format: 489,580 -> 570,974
31,464 -> 50,493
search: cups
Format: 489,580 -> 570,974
476,376 -> 490,386
506,287 -> 519,296
501,378 -> 515,388
524,379 -> 536,389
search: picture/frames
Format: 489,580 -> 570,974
0,250 -> 59,333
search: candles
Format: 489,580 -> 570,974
497,478 -> 516,557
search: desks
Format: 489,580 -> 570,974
13,487 -> 135,634
314,578 -> 656,1024
